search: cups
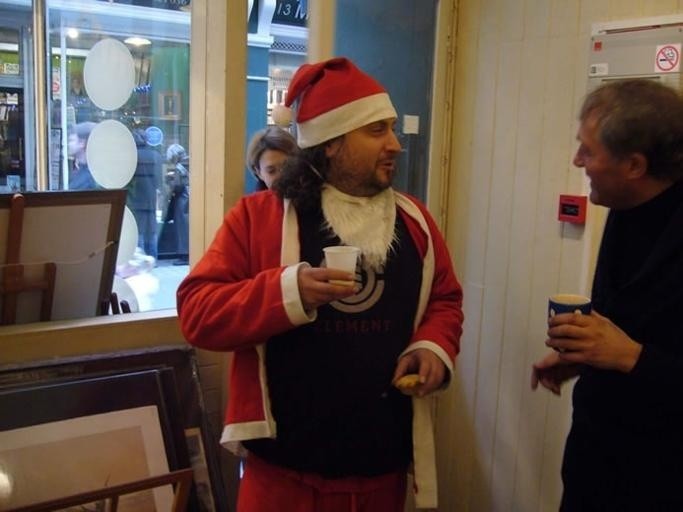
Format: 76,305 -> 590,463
547,292 -> 593,355
322,245 -> 359,288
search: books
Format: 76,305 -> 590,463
1,91 -> 25,178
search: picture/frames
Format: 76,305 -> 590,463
2,340 -> 229,511
0,188 -> 130,326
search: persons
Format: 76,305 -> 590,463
528,79 -> 682,512
70,77 -> 83,97
244,124 -> 300,193
173,56 -> 461,510
163,143 -> 189,264
129,127 -> 161,259
63,119 -> 108,192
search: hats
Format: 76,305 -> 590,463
274,57 -> 398,149
